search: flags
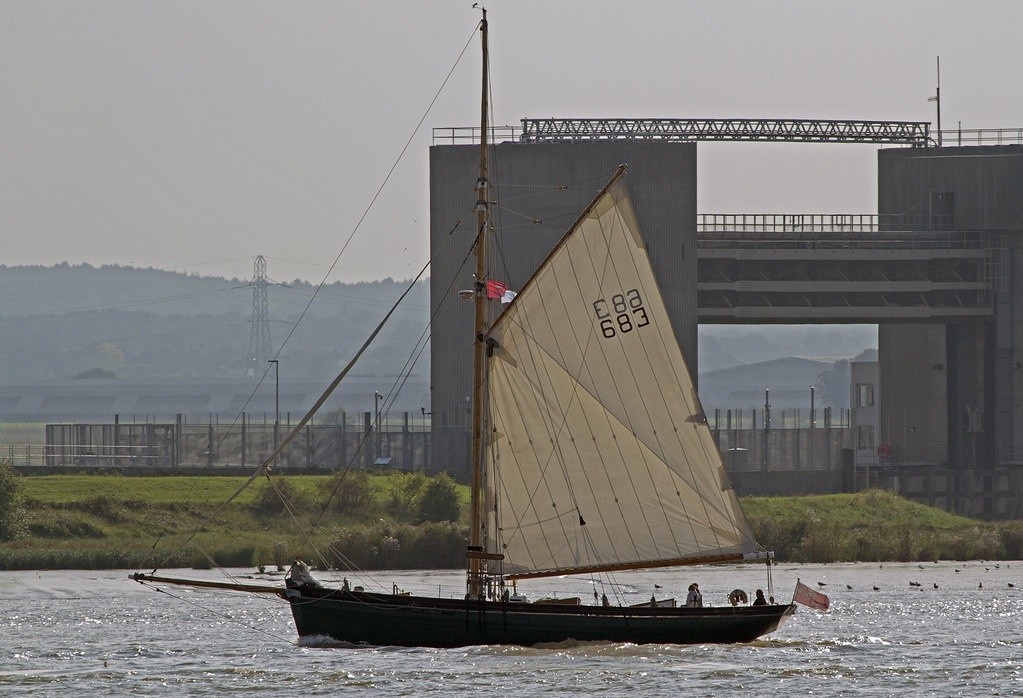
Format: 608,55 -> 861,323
794,582 -> 829,612
488,278 -> 507,301
500,290 -> 517,303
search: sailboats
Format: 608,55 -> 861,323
129,5 -> 834,645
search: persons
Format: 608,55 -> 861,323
685,583 -> 700,607
753,589 -> 766,605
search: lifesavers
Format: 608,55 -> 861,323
728,589 -> 747,607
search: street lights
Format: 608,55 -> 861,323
764,388 -> 770,429
374,391 -> 383,460
810,385 -> 815,430
268,359 -> 280,468
